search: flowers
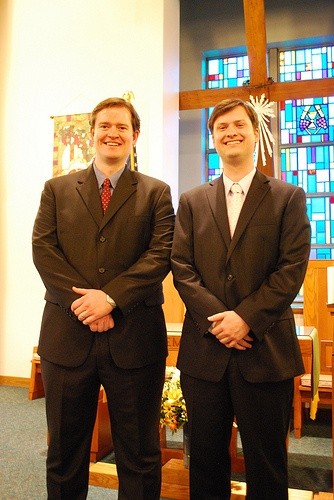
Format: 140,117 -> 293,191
162,375 -> 189,431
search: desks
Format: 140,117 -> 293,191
164,323 -> 321,437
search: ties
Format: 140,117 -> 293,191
228,183 -> 245,239
99,178 -> 111,214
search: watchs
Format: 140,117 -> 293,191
106,295 -> 116,308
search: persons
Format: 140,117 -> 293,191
167,99 -> 312,500
31,97 -> 176,500
